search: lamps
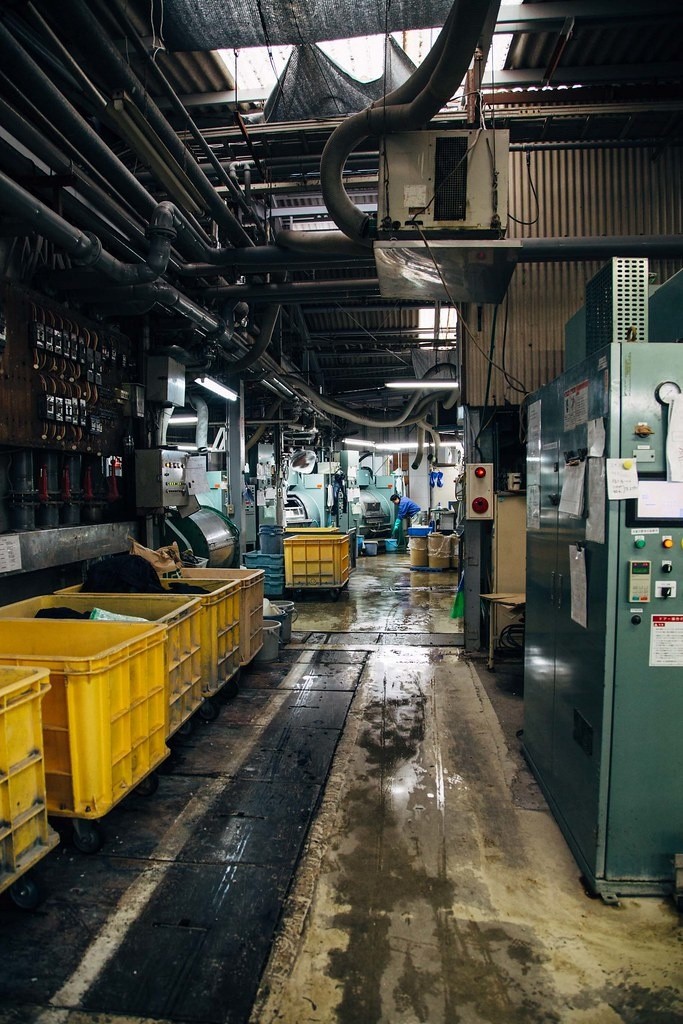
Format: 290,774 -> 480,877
385,380 -> 458,388
168,414 -> 197,423
177,444 -> 218,451
194,374 -> 238,402
344,438 -> 461,450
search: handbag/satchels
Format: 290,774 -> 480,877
129,537 -> 180,572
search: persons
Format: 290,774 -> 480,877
390,494 -> 421,551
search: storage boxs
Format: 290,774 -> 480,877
0,664 -> 62,910
264,582 -> 285,595
0,595 -> 206,766
242,564 -> 285,574
242,550 -> 284,566
53,579 -> 240,722
257,524 -> 282,554
158,569 -> 265,700
264,573 -> 285,582
283,527 -> 356,602
0,617 -> 170,854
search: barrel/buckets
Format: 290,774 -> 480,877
428,534 -> 449,567
410,536 -> 427,566
453,536 -> 460,567
385,539 -> 398,551
357,535 -> 364,551
362,541 -> 378,555
262,609 -> 288,645
266,601 -> 298,644
262,620 -> 284,660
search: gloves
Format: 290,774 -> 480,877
392,519 -> 400,534
